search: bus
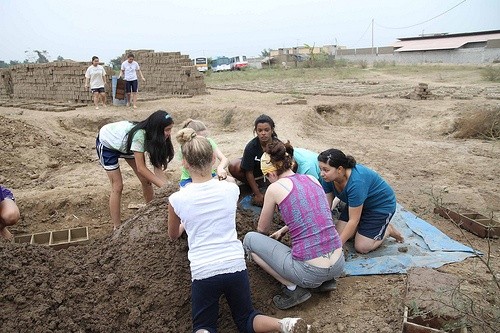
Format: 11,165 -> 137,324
210,57 -> 231,72
230,55 -> 248,70
192,58 -> 208,74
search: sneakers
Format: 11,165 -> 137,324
272,283 -> 311,309
309,277 -> 337,292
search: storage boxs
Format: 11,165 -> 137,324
434,202 -> 500,237
402,305 -> 468,333
11,226 -> 89,250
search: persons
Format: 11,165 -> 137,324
168,127 -> 317,333
293,148 -> 341,211
96,110 -> 175,228
85,56 -> 107,110
242,141 -> 345,309
228,114 -> 284,206
177,118 -> 230,188
0,185 -> 20,242
118,54 -> 145,108
317,148 -> 404,254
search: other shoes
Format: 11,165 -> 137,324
280,316 -> 307,333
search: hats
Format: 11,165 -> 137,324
260,152 -> 290,174
